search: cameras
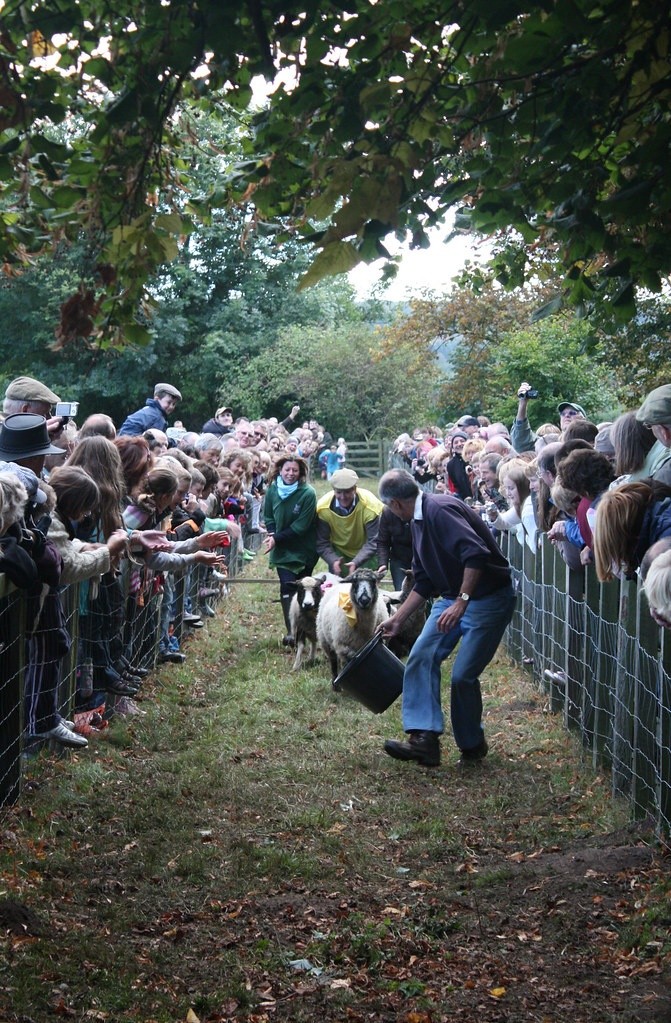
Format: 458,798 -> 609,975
55,402 -> 79,416
417,459 -> 426,465
486,502 -> 498,513
519,391 -> 538,400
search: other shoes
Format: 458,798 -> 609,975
108,666 -> 146,699
164,574 -> 226,666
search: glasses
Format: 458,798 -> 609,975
254,432 -> 265,438
560,411 -> 578,415
82,511 -> 91,517
536,470 -> 543,478
538,434 -> 543,436
242,432 -> 253,438
643,423 -> 654,429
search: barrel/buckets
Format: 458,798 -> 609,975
331,628 -> 407,715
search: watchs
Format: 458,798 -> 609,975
458,592 -> 471,601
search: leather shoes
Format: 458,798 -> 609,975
456,740 -> 488,766
385,731 -> 440,766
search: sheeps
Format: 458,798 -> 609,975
382,593 -> 401,616
285,571 -> 343,673
316,565 -> 388,692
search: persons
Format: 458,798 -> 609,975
394,382 -> 671,684
374,469 -> 517,766
379,503 -> 412,591
263,454 -> 316,642
117,383 -> 182,436
0,377 -> 347,747
316,469 -> 388,578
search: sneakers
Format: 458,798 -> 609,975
34,717 -> 75,736
47,721 -> 88,748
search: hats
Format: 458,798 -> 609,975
287,437 -> 298,444
499,434 -> 511,442
558,402 -> 586,417
398,442 -> 405,453
215,406 -> 233,418
594,428 -> 615,455
330,468 -> 359,492
195,433 -> 223,451
444,415 -> 480,440
636,383 -> 671,422
154,383 -> 182,402
0,377 -> 67,463
488,423 -> 508,440
393,433 -> 409,455
